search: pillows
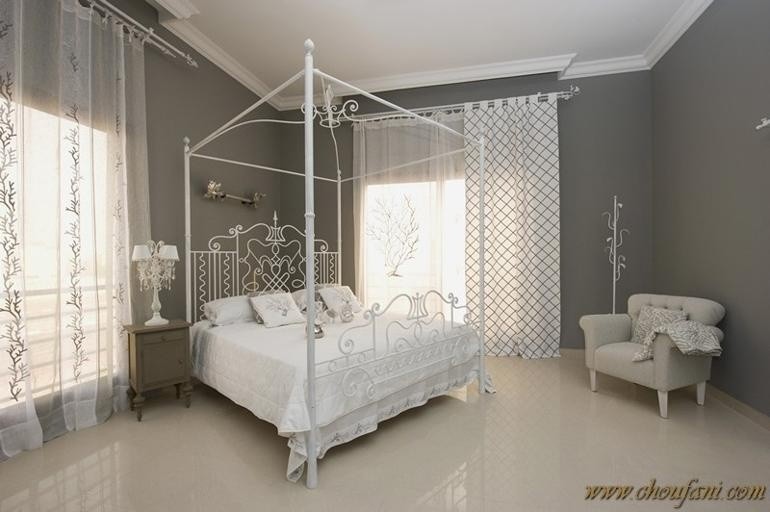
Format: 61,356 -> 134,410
629,303 -> 689,345
204,282 -> 362,328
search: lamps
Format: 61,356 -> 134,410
203,180 -> 266,208
130,240 -> 179,325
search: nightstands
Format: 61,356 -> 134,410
122,320 -> 194,422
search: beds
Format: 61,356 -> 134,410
183,38 -> 485,491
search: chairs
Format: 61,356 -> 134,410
579,293 -> 725,420
